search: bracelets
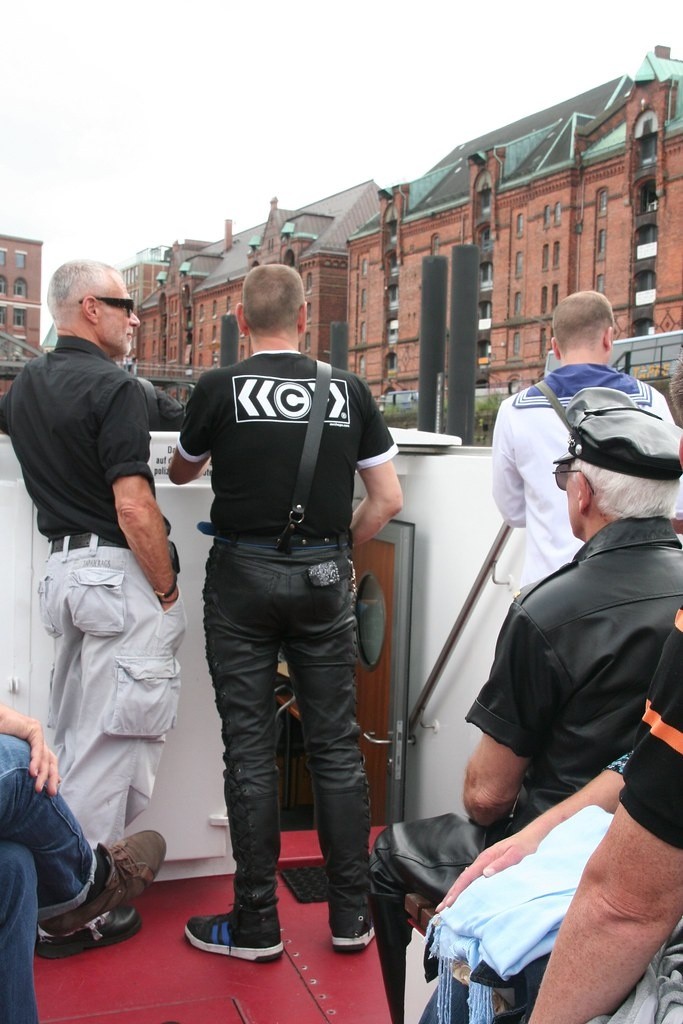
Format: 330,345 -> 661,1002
160,595 -> 178,603
154,575 -> 177,596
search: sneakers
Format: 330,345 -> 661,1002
184,910 -> 285,962
331,921 -> 372,950
40,828 -> 165,936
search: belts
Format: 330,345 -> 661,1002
198,518 -> 351,549
51,531 -> 122,552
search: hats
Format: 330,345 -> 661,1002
551,386 -> 683,482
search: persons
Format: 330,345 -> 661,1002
166,263 -> 405,964
367,354 -> 683,1024
0,703 -> 166,1024
492,291 -> 683,588
123,352 -> 137,374
0,261 -> 186,961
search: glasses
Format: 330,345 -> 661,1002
554,463 -> 598,496
80,295 -> 136,318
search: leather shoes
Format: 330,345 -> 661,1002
35,907 -> 141,959
